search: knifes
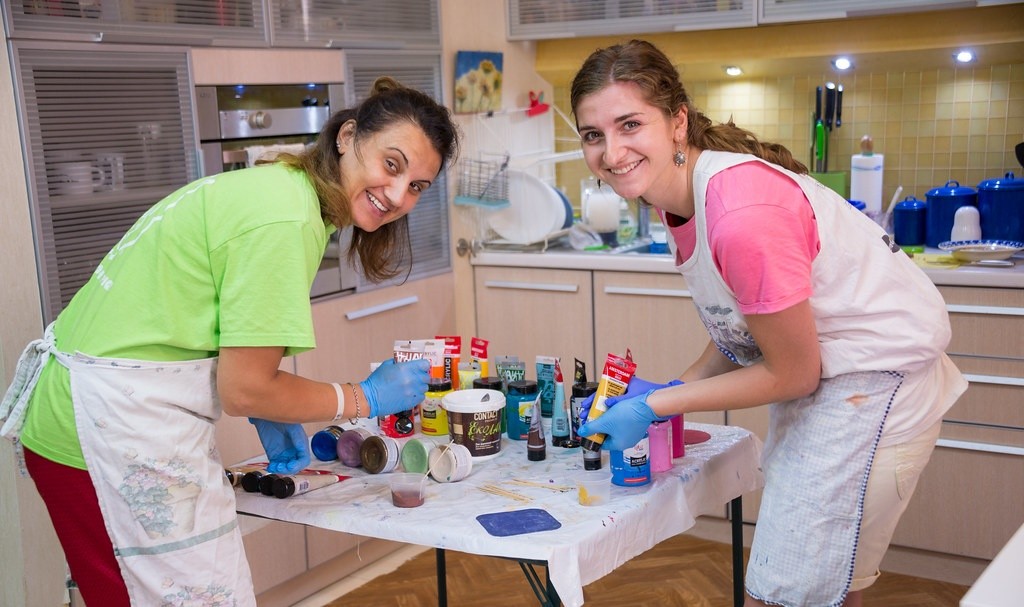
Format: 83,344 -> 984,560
809,82 -> 843,173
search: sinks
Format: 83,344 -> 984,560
618,241 -> 672,257
547,233 -> 635,253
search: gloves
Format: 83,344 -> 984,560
579,376 -> 687,426
577,389 -> 673,452
358,358 -> 430,420
249,411 -> 311,475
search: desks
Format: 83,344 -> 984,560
226,409 -> 766,607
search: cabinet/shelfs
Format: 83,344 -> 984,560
728,284 -> 1024,587
502,0 -> 1022,42
0,0 -> 452,341
472,265 -> 728,543
0,273 -> 453,607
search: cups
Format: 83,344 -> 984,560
96,155 -> 125,192
56,162 -> 105,196
581,178 -> 619,248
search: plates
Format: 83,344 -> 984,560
938,240 -> 1024,261
487,170 -> 572,242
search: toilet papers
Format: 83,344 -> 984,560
850,154 -> 884,212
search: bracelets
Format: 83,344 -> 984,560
331,381 -> 360,426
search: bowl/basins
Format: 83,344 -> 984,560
976,172 -> 1024,243
893,196 -> 926,246
388,473 -> 428,509
574,471 -> 613,506
925,180 -> 978,248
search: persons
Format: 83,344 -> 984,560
570,38 -> 970,607
0,76 -> 464,606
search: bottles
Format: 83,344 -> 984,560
380,408 -> 415,438
506,380 -> 539,441
419,378 -> 454,436
570,382 -> 600,442
619,198 -> 635,244
231,86 -> 247,110
473,377 -> 507,433
847,200 -> 866,215
302,84 -> 319,108
610,413 -> 685,487
